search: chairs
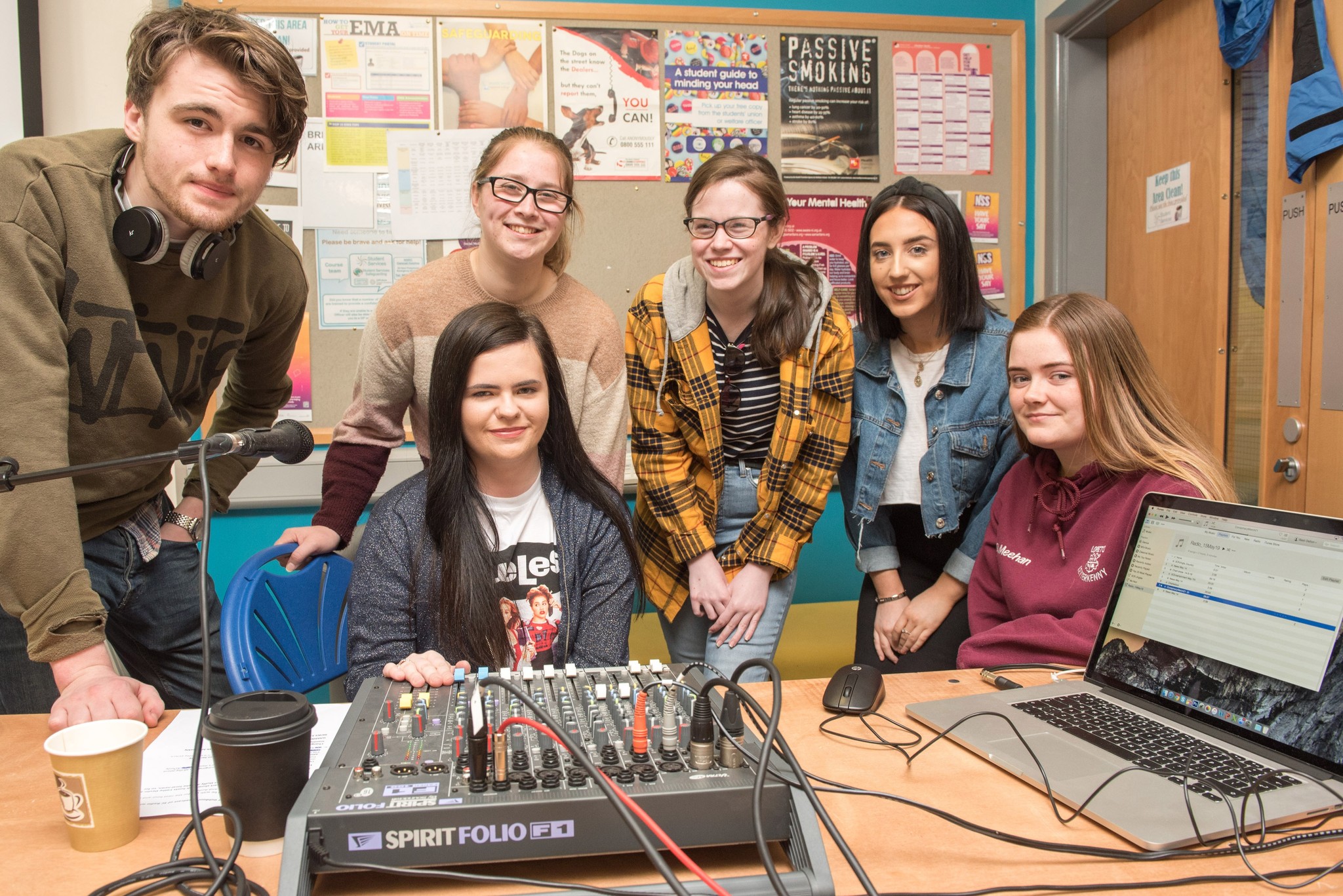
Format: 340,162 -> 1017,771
219,544 -> 357,699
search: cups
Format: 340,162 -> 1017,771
201,689 -> 318,858
44,719 -> 148,852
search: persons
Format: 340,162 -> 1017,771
835,175 -> 1032,674
623,156 -> 857,683
275,127 -> 635,578
1,3 -> 310,733
954,292 -> 1239,668
347,302 -> 646,702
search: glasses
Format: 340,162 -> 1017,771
719,341 -> 747,416
476,176 -> 576,214
683,211 -> 777,240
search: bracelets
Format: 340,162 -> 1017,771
875,589 -> 907,604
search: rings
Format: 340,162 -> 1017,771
902,628 -> 911,635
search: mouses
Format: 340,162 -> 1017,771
823,663 -> 886,717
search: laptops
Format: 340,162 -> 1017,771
904,489 -> 1343,854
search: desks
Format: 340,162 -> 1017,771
0,666 -> 1343,896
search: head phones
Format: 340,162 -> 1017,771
109,144 -> 240,278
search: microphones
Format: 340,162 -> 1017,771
212,419 -> 316,464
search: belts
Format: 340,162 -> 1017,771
724,459 -> 763,469
